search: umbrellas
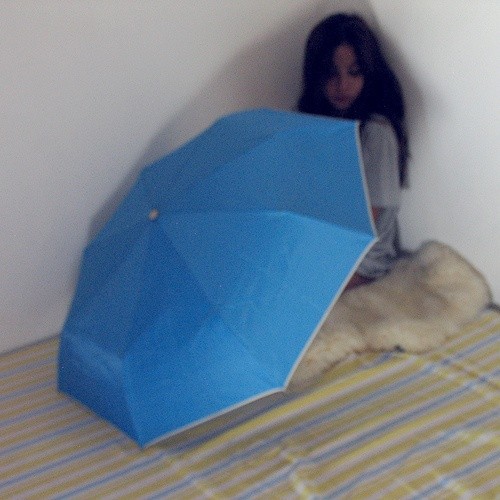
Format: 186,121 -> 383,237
56,105 -> 381,452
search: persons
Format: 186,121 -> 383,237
291,11 -> 408,292
